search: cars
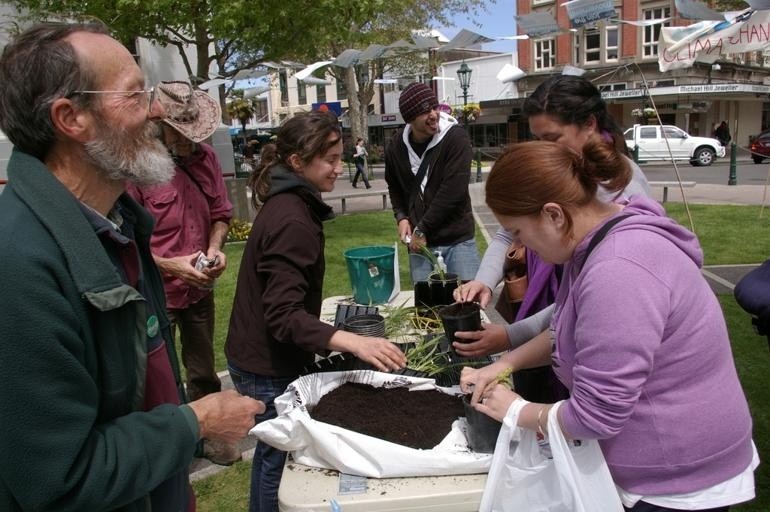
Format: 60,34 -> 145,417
749,128 -> 770,164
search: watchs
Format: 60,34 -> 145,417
412,226 -> 424,239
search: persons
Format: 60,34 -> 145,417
223,110 -> 409,512
452,74 -> 651,400
122,80 -> 236,401
0,24 -> 267,511
715,121 -> 732,147
351,138 -> 373,190
383,81 -> 481,310
460,141 -> 762,511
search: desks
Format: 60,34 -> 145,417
274,288 -> 518,510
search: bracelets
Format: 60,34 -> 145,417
538,404 -> 546,437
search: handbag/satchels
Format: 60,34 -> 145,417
502,234 -> 531,320
407,146 -> 435,214
578,212 -> 641,269
479,398 -> 627,511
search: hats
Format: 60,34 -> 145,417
398,80 -> 440,124
150,80 -> 224,145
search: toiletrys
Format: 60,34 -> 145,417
434,249 -> 447,273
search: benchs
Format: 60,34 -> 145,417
646,181 -> 699,207
322,187 -> 391,217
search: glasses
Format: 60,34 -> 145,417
72,85 -> 160,102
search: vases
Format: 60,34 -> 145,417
339,312 -> 386,339
436,301 -> 484,343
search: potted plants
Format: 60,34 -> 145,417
410,239 -> 463,286
460,370 -> 515,458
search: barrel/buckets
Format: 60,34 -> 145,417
343,314 -> 385,368
343,246 -> 396,306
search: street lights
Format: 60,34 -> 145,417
456,55 -> 475,131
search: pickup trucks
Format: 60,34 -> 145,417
621,122 -> 727,166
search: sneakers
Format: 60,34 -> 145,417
351,182 -> 373,190
198,436 -> 242,466
